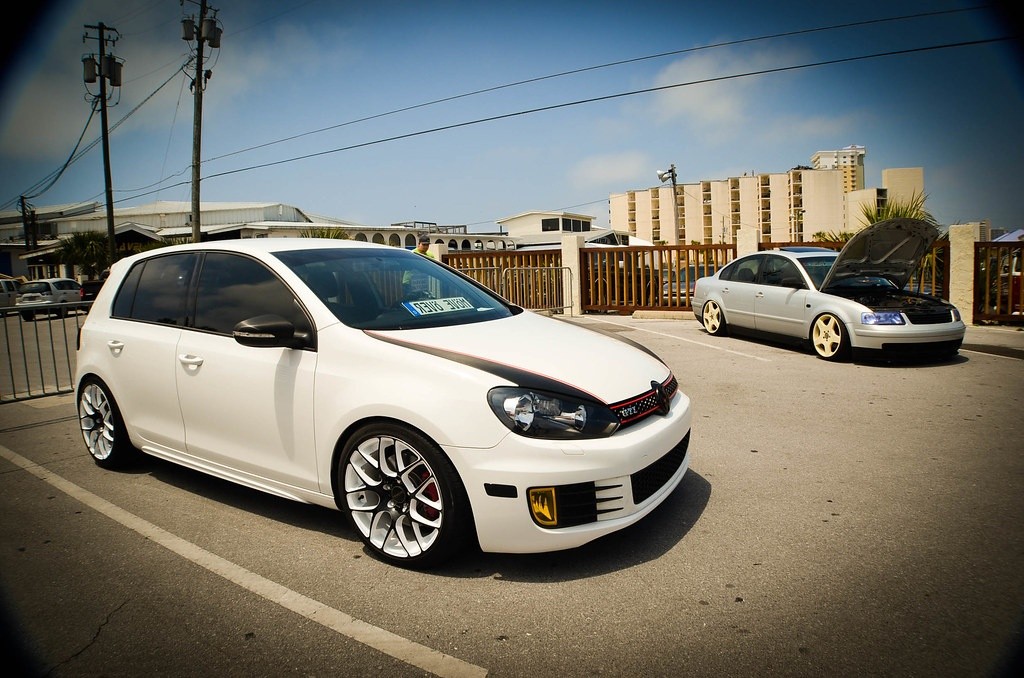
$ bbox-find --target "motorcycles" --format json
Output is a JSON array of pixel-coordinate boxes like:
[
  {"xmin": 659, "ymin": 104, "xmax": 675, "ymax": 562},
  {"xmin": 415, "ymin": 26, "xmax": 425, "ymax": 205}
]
[{"xmin": 992, "ymin": 248, "xmax": 1023, "ymax": 327}]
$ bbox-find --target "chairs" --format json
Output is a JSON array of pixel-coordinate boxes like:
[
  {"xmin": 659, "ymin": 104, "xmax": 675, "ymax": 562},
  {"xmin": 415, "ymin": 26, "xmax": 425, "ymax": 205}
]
[
  {"xmin": 738, "ymin": 268, "xmax": 755, "ymax": 283},
  {"xmin": 306, "ymin": 267, "xmax": 354, "ymax": 325}
]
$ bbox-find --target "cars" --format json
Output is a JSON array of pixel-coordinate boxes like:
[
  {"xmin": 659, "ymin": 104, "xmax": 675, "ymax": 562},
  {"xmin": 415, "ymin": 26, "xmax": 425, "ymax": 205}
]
[
  {"xmin": 74, "ymin": 235, "xmax": 695, "ymax": 575},
  {"xmin": 14, "ymin": 278, "xmax": 82, "ymax": 322},
  {"xmin": 0, "ymin": 278, "xmax": 22, "ymax": 317},
  {"xmin": 690, "ymin": 217, "xmax": 966, "ymax": 364}
]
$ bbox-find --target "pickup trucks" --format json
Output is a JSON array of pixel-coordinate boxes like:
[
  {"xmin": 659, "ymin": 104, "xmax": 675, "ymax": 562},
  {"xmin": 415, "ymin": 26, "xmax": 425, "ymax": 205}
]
[{"xmin": 80, "ymin": 268, "xmax": 111, "ymax": 313}]
